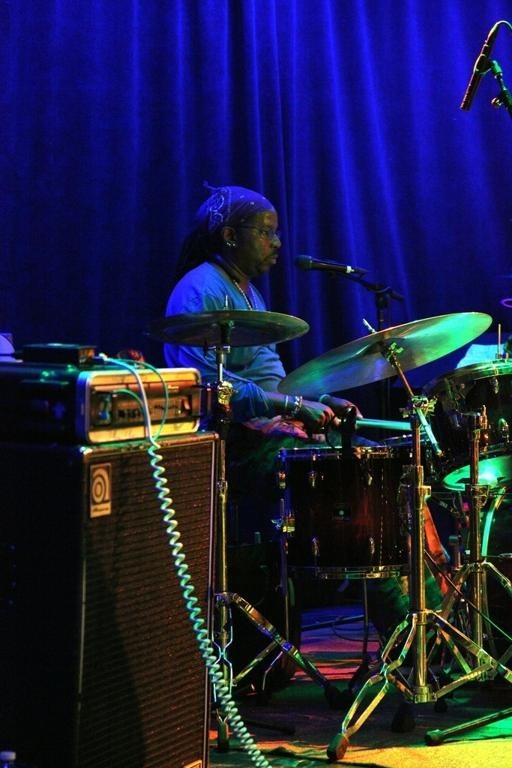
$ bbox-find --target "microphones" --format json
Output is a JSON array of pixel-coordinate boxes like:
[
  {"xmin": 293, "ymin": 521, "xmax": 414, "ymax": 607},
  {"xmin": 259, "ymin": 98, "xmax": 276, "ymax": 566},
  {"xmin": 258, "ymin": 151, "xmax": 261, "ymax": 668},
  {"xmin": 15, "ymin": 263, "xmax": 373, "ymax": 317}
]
[
  {"xmin": 294, "ymin": 253, "xmax": 352, "ymax": 281},
  {"xmin": 457, "ymin": 29, "xmax": 503, "ymax": 113}
]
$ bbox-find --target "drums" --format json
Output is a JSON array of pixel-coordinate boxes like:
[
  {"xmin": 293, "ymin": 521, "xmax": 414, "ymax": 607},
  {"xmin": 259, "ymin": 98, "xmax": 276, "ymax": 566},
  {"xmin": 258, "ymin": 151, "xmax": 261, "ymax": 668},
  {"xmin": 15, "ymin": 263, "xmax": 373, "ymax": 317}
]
[
  {"xmin": 442, "ymin": 455, "xmax": 511, "ymax": 653},
  {"xmin": 421, "ymin": 358, "xmax": 512, "ymax": 446},
  {"xmin": 277, "ymin": 445, "xmax": 412, "ymax": 582}
]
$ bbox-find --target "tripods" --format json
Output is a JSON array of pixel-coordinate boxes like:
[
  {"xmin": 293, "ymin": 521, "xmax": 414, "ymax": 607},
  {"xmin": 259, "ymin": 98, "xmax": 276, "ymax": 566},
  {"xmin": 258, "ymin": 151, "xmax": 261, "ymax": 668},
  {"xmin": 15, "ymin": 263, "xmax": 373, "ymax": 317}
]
[
  {"xmin": 329, "ymin": 423, "xmax": 511, "ymax": 762},
  {"xmin": 391, "ymin": 423, "xmax": 511, "ymax": 734},
  {"xmin": 213, "ymin": 432, "xmax": 343, "ymax": 751}
]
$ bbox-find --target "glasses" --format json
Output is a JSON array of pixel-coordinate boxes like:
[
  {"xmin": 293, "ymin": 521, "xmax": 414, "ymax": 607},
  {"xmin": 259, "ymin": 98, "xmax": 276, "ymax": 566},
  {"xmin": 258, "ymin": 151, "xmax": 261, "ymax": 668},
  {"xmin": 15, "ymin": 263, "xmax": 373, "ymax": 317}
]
[{"xmin": 223, "ymin": 222, "xmax": 283, "ymax": 241}]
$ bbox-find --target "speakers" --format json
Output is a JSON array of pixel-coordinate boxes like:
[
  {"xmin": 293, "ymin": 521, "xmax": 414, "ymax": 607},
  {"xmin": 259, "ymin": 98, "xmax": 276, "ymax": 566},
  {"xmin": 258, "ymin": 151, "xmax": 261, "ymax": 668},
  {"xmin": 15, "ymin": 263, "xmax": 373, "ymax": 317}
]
[{"xmin": 0, "ymin": 430, "xmax": 222, "ymax": 767}]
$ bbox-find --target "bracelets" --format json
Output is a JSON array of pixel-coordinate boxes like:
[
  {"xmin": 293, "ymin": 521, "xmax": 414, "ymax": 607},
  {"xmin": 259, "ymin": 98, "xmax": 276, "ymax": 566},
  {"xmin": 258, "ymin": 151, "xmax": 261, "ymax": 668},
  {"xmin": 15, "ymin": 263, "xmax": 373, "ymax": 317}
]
[
  {"xmin": 282, "ymin": 395, "xmax": 303, "ymax": 421},
  {"xmin": 319, "ymin": 393, "xmax": 330, "ymax": 404}
]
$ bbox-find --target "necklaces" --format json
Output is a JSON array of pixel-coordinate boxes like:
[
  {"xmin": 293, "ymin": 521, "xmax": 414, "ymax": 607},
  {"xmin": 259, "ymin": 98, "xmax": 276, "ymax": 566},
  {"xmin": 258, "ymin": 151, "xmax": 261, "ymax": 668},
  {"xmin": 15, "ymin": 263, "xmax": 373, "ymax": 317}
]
[{"xmin": 232, "ymin": 279, "xmax": 257, "ymax": 311}]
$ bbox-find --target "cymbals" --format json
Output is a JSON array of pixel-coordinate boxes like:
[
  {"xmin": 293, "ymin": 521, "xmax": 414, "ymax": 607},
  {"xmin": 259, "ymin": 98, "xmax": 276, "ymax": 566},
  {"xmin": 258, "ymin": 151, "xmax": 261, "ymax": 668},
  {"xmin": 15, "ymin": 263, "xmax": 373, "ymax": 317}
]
[
  {"xmin": 277, "ymin": 312, "xmax": 492, "ymax": 395},
  {"xmin": 149, "ymin": 309, "xmax": 309, "ymax": 348}
]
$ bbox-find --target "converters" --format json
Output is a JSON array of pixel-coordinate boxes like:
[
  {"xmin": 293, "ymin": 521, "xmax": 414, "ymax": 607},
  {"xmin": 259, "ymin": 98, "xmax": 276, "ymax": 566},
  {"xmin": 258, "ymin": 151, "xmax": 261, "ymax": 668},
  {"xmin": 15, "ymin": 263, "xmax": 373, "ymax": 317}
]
[{"xmin": 24, "ymin": 340, "xmax": 99, "ymax": 368}]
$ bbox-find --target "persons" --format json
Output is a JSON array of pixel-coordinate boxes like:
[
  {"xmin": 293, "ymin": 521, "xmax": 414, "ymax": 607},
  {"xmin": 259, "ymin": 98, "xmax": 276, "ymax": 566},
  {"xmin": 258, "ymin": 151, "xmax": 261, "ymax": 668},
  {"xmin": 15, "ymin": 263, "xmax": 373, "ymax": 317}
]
[{"xmin": 161, "ymin": 185, "xmax": 467, "ymax": 690}]
